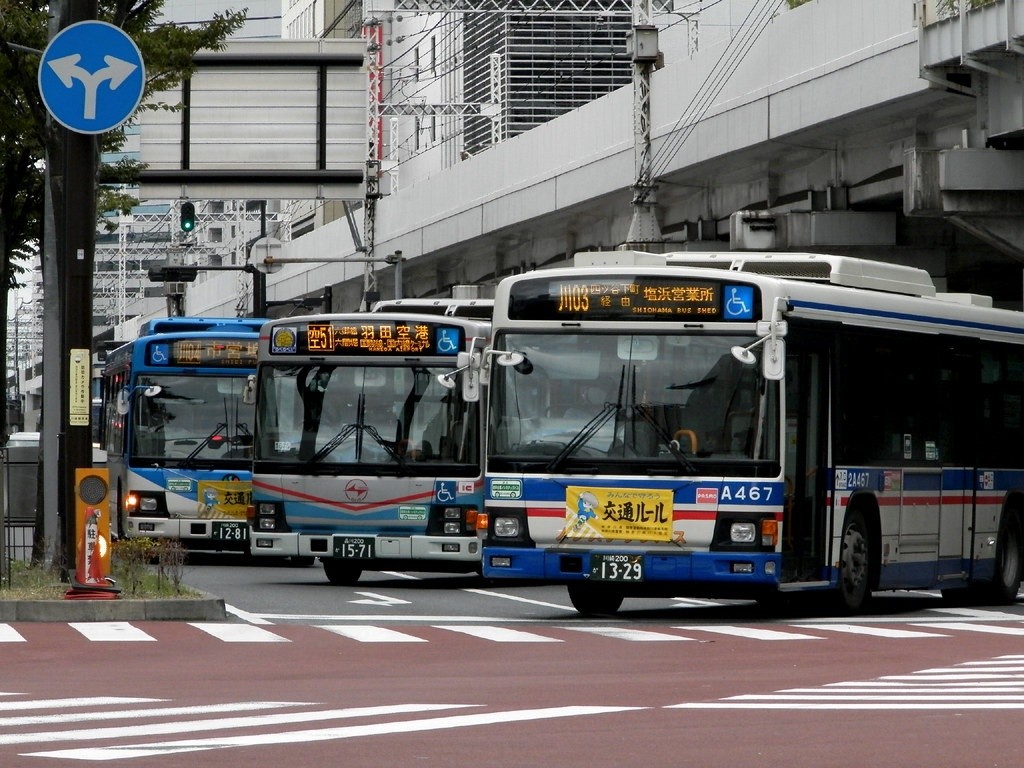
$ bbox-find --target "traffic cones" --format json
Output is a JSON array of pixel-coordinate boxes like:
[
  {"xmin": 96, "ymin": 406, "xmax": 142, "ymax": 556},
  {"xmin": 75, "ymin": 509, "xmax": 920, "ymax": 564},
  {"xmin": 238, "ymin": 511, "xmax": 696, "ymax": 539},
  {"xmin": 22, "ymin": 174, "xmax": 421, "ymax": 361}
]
[{"xmin": 65, "ymin": 506, "xmax": 117, "ymax": 599}]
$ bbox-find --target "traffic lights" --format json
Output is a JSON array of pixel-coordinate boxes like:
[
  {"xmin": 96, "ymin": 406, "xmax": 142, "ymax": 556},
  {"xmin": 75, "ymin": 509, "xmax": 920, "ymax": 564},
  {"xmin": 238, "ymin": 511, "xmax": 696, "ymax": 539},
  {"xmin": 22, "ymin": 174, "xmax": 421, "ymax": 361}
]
[{"xmin": 180, "ymin": 202, "xmax": 195, "ymax": 230}]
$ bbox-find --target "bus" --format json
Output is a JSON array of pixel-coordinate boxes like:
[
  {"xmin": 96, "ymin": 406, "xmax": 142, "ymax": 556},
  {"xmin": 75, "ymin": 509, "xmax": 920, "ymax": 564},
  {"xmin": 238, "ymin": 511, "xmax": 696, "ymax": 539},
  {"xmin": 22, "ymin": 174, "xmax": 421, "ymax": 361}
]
[
  {"xmin": 251, "ymin": 295, "xmax": 502, "ymax": 586},
  {"xmin": 97, "ymin": 316, "xmax": 273, "ymax": 556},
  {"xmin": 478, "ymin": 240, "xmax": 1024, "ymax": 617}
]
